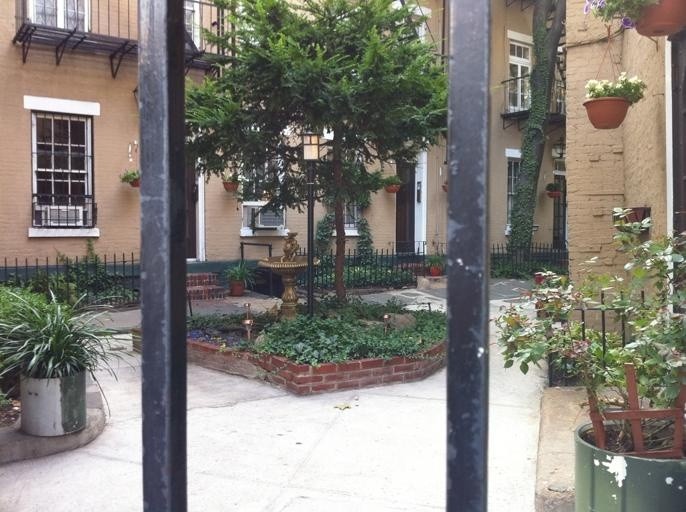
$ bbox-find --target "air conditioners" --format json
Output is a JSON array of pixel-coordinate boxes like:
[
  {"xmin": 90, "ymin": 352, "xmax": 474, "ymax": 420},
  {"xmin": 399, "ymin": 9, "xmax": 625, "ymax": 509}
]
[
  {"xmin": 41, "ymin": 206, "xmax": 83, "ymax": 227},
  {"xmin": 249, "ymin": 206, "xmax": 286, "ymax": 229}
]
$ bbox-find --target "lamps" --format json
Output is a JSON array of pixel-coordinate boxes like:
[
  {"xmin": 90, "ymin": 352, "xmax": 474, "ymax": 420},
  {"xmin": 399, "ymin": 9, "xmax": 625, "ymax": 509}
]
[{"xmin": 551, "ymin": 137, "xmax": 565, "ymax": 160}]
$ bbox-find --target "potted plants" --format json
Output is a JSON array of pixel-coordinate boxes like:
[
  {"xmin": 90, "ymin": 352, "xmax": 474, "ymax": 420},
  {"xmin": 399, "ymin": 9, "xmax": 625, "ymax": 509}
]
[
  {"xmin": 497, "ymin": 209, "xmax": 686, "ymax": 512},
  {"xmin": 545, "ymin": 183, "xmax": 561, "ymax": 198},
  {"xmin": 121, "ymin": 171, "xmax": 140, "ymax": 187},
  {"xmin": 384, "ymin": 175, "xmax": 401, "ymax": 193},
  {"xmin": 429, "ymin": 256, "xmax": 444, "ymax": 276},
  {"xmin": 0, "ymin": 287, "xmax": 141, "ymax": 436},
  {"xmin": 223, "ymin": 260, "xmax": 254, "ymax": 296},
  {"xmin": 221, "ymin": 173, "xmax": 241, "ymax": 192}
]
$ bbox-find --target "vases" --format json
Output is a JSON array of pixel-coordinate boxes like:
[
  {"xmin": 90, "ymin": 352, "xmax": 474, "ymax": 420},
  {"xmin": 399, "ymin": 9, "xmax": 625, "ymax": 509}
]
[
  {"xmin": 582, "ymin": 97, "xmax": 633, "ymax": 129},
  {"xmin": 634, "ymin": 0, "xmax": 686, "ymax": 36}
]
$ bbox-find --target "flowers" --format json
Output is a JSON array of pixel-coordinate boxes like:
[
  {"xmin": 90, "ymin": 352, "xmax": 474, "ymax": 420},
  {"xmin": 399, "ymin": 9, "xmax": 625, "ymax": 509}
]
[
  {"xmin": 584, "ymin": 0, "xmax": 661, "ymax": 29},
  {"xmin": 585, "ymin": 72, "xmax": 648, "ymax": 103}
]
